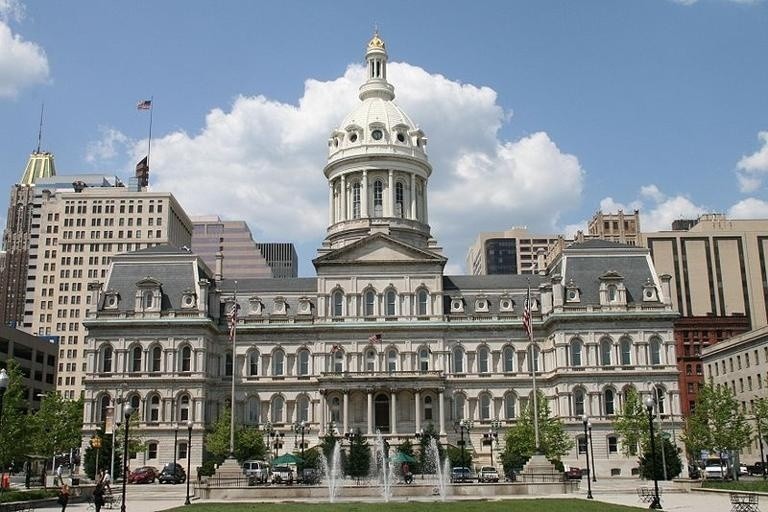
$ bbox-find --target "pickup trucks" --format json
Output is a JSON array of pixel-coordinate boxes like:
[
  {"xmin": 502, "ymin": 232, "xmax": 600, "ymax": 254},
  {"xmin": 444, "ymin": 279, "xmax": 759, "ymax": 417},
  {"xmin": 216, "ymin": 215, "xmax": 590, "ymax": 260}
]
[{"xmin": 452, "ymin": 465, "xmax": 499, "ymax": 483}]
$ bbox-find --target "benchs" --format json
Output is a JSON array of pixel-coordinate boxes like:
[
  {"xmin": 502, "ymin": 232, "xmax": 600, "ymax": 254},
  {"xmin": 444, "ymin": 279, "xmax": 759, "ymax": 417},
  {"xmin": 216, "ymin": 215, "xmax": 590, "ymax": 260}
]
[
  {"xmin": 636, "ymin": 485, "xmax": 663, "ymax": 504},
  {"xmin": 729, "ymin": 490, "xmax": 761, "ymax": 512}
]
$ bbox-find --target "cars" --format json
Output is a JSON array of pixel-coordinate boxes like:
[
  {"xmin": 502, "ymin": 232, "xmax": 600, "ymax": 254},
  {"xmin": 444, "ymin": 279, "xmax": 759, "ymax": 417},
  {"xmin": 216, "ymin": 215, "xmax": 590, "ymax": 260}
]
[
  {"xmin": 243, "ymin": 460, "xmax": 323, "ymax": 484},
  {"xmin": 128, "ymin": 463, "xmax": 186, "ymax": 484},
  {"xmin": 688, "ymin": 456, "xmax": 768, "ymax": 479},
  {"xmin": 565, "ymin": 467, "xmax": 582, "ymax": 479}
]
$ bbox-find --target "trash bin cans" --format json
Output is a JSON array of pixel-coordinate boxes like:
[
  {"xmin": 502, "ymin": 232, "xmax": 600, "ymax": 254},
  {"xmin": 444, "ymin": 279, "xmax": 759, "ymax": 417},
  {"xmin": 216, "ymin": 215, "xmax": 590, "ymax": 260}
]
[{"xmin": 72, "ymin": 478, "xmax": 79, "ymax": 485}]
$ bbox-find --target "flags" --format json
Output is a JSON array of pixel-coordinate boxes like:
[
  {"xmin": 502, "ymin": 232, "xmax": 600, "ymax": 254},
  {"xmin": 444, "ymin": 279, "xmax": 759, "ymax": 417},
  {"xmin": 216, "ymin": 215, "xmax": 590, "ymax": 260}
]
[
  {"xmin": 523, "ymin": 284, "xmax": 531, "ymax": 339},
  {"xmin": 370, "ymin": 333, "xmax": 381, "ymax": 344},
  {"xmin": 137, "ymin": 100, "xmax": 151, "ymax": 109},
  {"xmin": 229, "ymin": 288, "xmax": 234, "ymax": 346}
]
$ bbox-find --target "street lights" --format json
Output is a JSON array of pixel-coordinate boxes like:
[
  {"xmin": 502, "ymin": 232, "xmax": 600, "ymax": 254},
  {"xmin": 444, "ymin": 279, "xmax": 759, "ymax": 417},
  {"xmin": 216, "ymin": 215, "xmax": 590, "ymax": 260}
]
[
  {"xmin": 645, "ymin": 397, "xmax": 661, "ymax": 510},
  {"xmin": 300, "ymin": 419, "xmax": 306, "ymax": 481},
  {"xmin": 489, "ymin": 428, "xmax": 493, "ymax": 466},
  {"xmin": 582, "ymin": 414, "xmax": 596, "ymax": 499},
  {"xmin": 349, "ymin": 428, "xmax": 354, "ymax": 479},
  {"xmin": 173, "ymin": 423, "xmax": 179, "ymax": 484},
  {"xmin": 459, "ymin": 419, "xmax": 466, "ymax": 483},
  {"xmin": 0, "ymin": 368, "xmax": 9, "ymax": 413},
  {"xmin": 185, "ymin": 420, "xmax": 193, "ymax": 505},
  {"xmin": 121, "ymin": 402, "xmax": 134, "ymax": 512}
]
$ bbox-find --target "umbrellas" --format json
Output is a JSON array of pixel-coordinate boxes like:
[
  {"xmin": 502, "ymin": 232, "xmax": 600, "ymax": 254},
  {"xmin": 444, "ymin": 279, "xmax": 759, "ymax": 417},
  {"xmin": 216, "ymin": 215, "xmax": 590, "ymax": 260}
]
[
  {"xmin": 388, "ymin": 453, "xmax": 421, "ymax": 465},
  {"xmin": 273, "ymin": 453, "xmax": 304, "ymax": 466}
]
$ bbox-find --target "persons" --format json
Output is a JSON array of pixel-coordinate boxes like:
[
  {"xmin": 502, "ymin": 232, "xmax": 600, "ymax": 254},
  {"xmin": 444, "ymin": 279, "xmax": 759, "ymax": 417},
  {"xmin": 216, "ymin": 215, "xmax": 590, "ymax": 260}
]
[
  {"xmin": 60, "ymin": 483, "xmax": 70, "ymax": 512},
  {"xmin": 2, "ymin": 471, "xmax": 10, "ymax": 489},
  {"xmin": 93, "ymin": 482, "xmax": 106, "ymax": 512},
  {"xmin": 402, "ymin": 462, "xmax": 411, "ymax": 483},
  {"xmin": 102, "ymin": 469, "xmax": 110, "ymax": 483}
]
